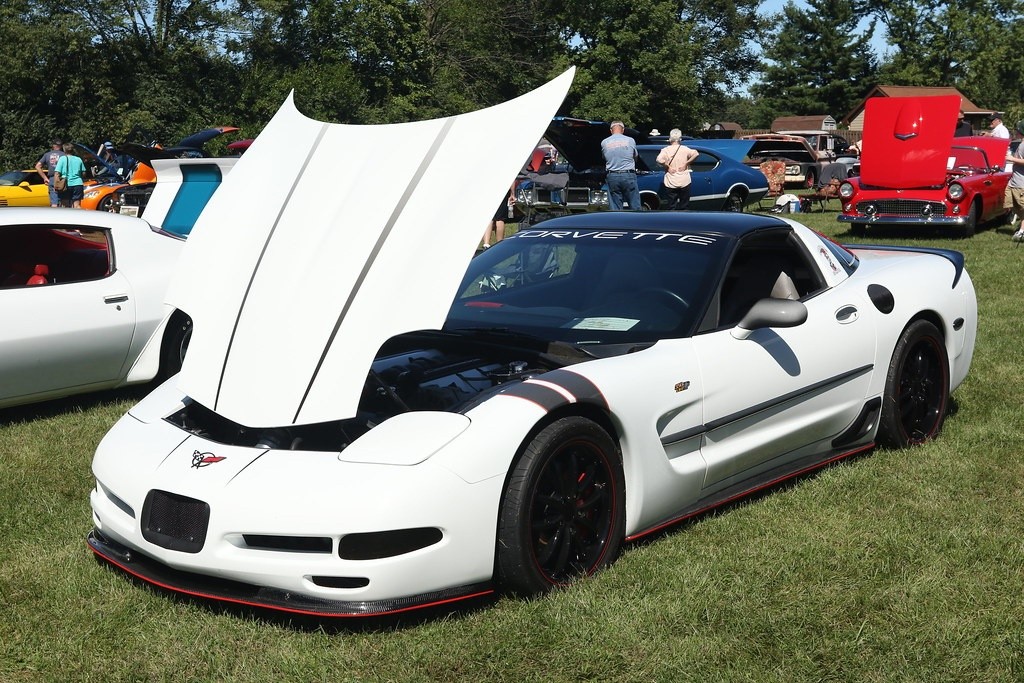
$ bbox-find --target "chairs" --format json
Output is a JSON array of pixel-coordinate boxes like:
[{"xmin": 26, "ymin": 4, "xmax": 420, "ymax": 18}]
[
  {"xmin": 718, "ymin": 240, "xmax": 800, "ymax": 325},
  {"xmin": 799, "ymin": 163, "xmax": 846, "ymax": 214},
  {"xmin": 0, "ymin": 261, "xmax": 49, "ymax": 286},
  {"xmin": 757, "ymin": 161, "xmax": 786, "ymax": 211}
]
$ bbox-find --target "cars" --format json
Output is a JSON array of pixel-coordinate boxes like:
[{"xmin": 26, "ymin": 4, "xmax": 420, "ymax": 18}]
[
  {"xmin": 836, "ymin": 96, "xmax": 1018, "ymax": 236},
  {"xmin": 514, "ymin": 116, "xmax": 771, "ymax": 213},
  {"xmin": 748, "ymin": 129, "xmax": 862, "ymax": 189}
]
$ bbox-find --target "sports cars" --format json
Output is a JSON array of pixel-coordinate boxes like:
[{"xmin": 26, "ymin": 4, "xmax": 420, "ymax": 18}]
[
  {"xmin": 0, "ymin": 168, "xmax": 51, "ymax": 208},
  {"xmin": 78, "ymin": 139, "xmax": 164, "ymax": 214},
  {"xmin": 1, "ymin": 158, "xmax": 243, "ymax": 416},
  {"xmin": 88, "ymin": 65, "xmax": 983, "ymax": 625}
]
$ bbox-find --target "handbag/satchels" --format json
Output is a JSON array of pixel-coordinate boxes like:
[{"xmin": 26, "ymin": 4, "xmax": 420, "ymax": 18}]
[
  {"xmin": 658, "ymin": 182, "xmax": 667, "ymax": 200},
  {"xmin": 54, "ymin": 178, "xmax": 67, "ymax": 192}
]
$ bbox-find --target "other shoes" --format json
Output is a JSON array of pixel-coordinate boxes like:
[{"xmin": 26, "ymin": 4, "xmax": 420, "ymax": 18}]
[
  {"xmin": 482, "ymin": 245, "xmax": 490, "ymax": 252},
  {"xmin": 1013, "ymin": 230, "xmax": 1024, "ymax": 240}
]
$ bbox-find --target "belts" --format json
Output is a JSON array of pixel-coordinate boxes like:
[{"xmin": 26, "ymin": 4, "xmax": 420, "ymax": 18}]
[{"xmin": 606, "ymin": 170, "xmax": 635, "ymax": 174}]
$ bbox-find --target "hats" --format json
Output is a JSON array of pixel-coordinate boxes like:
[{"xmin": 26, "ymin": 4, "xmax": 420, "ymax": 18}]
[
  {"xmin": 105, "ymin": 142, "xmax": 113, "ymax": 149},
  {"xmin": 987, "ymin": 112, "xmax": 1001, "ymax": 121},
  {"xmin": 52, "ymin": 139, "xmax": 63, "ymax": 146},
  {"xmin": 1014, "ymin": 118, "xmax": 1024, "ymax": 135}
]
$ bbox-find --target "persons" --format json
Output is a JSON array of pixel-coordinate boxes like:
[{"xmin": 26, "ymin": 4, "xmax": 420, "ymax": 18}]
[
  {"xmin": 601, "ymin": 120, "xmax": 642, "ymax": 212},
  {"xmin": 35, "ymin": 139, "xmax": 87, "ymax": 209},
  {"xmin": 103, "ymin": 141, "xmax": 117, "ymax": 164},
  {"xmin": 1003, "ymin": 119, "xmax": 1024, "ymax": 242},
  {"xmin": 954, "ymin": 109, "xmax": 973, "ymax": 137},
  {"xmin": 980, "ymin": 111, "xmax": 1010, "ymax": 139},
  {"xmin": 656, "ymin": 129, "xmax": 700, "ymax": 211},
  {"xmin": 482, "ymin": 181, "xmax": 516, "ymax": 247}
]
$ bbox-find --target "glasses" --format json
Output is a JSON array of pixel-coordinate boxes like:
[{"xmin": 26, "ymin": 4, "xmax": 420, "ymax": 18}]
[{"xmin": 958, "ymin": 117, "xmax": 965, "ymax": 121}]
